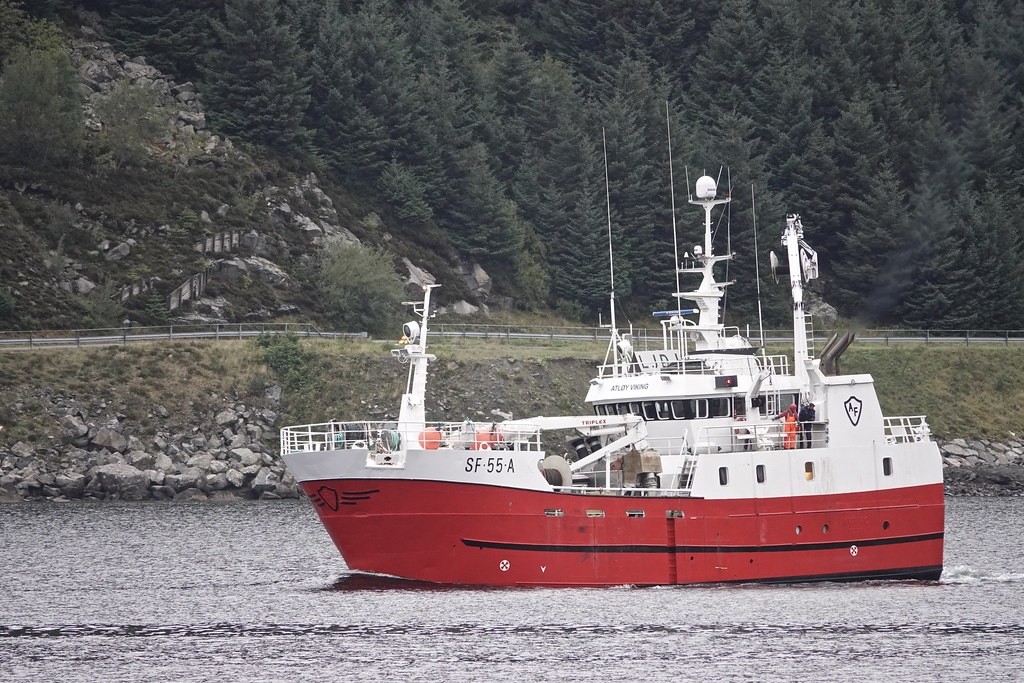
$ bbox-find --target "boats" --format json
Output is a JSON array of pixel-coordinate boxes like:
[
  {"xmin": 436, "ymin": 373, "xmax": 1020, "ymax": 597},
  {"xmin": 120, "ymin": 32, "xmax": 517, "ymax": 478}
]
[{"xmin": 279, "ymin": 99, "xmax": 949, "ymax": 586}]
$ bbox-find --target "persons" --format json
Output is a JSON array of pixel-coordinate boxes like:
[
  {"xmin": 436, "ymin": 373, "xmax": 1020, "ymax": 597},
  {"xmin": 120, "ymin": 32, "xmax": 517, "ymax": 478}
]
[
  {"xmin": 770, "ymin": 403, "xmax": 800, "ymax": 450},
  {"xmin": 799, "ymin": 403, "xmax": 816, "ymax": 449}
]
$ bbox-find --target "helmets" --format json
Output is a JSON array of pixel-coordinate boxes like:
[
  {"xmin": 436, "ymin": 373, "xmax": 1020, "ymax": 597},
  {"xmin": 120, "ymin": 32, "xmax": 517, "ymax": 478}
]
[{"xmin": 790, "ymin": 404, "xmax": 797, "ymax": 409}]
